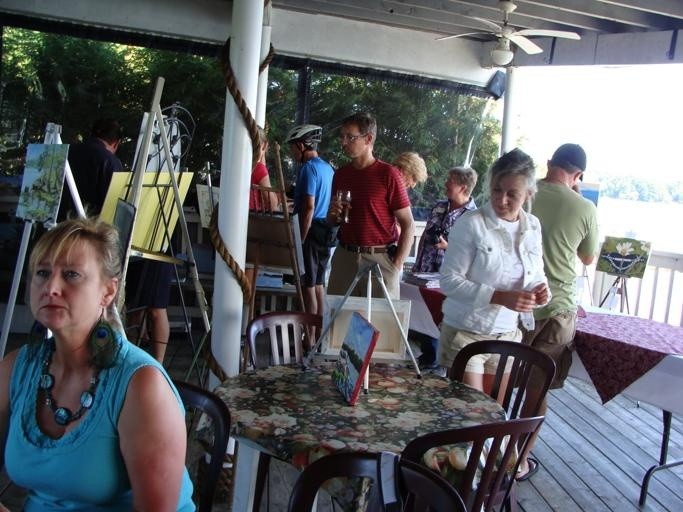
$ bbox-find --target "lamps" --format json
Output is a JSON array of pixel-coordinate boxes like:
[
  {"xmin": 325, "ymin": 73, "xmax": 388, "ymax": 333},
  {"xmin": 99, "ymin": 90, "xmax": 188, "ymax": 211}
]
[{"xmin": 489, "ymin": 39, "xmax": 515, "ymax": 66}]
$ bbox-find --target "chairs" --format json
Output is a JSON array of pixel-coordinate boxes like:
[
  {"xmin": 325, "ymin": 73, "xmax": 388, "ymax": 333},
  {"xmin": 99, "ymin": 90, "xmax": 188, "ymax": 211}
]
[
  {"xmin": 400, "ymin": 414, "xmax": 544, "ymax": 512},
  {"xmin": 244, "ymin": 311, "xmax": 321, "ymax": 371},
  {"xmin": 449, "ymin": 340, "xmax": 556, "ymax": 512},
  {"xmin": 171, "ymin": 380, "xmax": 230, "ymax": 511},
  {"xmin": 286, "ymin": 450, "xmax": 466, "ymax": 512}
]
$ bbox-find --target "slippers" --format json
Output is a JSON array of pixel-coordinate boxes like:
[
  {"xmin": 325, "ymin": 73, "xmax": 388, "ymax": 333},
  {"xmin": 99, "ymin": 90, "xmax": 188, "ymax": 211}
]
[{"xmin": 515, "ymin": 457, "xmax": 539, "ymax": 481}]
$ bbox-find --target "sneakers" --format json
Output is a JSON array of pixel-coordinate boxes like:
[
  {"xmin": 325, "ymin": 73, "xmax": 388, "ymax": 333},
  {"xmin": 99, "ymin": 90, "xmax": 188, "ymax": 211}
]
[
  {"xmin": 430, "ymin": 367, "xmax": 448, "ymax": 378},
  {"xmin": 407, "ymin": 354, "xmax": 438, "ymax": 370}
]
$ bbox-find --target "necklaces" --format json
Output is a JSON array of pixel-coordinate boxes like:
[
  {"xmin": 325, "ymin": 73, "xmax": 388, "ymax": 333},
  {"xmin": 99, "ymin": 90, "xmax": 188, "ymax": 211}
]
[{"xmin": 38, "ymin": 337, "xmax": 102, "ymax": 425}]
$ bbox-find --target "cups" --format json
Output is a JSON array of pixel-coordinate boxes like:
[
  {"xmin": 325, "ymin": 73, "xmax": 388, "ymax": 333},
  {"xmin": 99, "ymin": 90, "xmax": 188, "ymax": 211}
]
[{"xmin": 335, "ymin": 190, "xmax": 352, "ymax": 225}]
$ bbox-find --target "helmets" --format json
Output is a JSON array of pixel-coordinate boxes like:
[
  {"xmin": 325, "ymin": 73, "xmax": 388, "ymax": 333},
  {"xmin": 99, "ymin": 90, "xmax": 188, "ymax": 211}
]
[{"xmin": 284, "ymin": 124, "xmax": 323, "ymax": 143}]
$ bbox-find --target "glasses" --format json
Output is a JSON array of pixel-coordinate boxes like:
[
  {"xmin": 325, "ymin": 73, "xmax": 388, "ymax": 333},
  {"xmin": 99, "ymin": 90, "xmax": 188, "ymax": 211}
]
[{"xmin": 339, "ymin": 131, "xmax": 371, "ymax": 142}]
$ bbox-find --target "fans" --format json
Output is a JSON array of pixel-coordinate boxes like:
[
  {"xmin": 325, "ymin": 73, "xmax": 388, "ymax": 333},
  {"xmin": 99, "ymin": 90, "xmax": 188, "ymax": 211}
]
[{"xmin": 434, "ymin": 0, "xmax": 581, "ymax": 55}]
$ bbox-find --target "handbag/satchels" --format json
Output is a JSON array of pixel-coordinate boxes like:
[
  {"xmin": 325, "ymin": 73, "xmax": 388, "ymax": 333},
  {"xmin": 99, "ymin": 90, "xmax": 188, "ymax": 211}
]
[{"xmin": 310, "ymin": 219, "xmax": 341, "ymax": 248}]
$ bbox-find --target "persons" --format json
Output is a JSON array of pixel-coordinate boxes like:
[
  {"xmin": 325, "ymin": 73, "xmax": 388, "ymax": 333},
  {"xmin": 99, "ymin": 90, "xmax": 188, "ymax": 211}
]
[
  {"xmin": 322, "ymin": 114, "xmax": 414, "ymax": 300},
  {"xmin": 0, "ymin": 218, "xmax": 197, "ymax": 512},
  {"xmin": 437, "ymin": 147, "xmax": 553, "ymax": 411},
  {"xmin": 59, "ymin": 115, "xmax": 123, "ymax": 219},
  {"xmin": 511, "ymin": 144, "xmax": 596, "ymax": 482},
  {"xmin": 282, "ymin": 124, "xmax": 338, "ymax": 355},
  {"xmin": 249, "ymin": 126, "xmax": 278, "ymax": 211},
  {"xmin": 391, "ymin": 152, "xmax": 428, "ymax": 189},
  {"xmin": 411, "ymin": 166, "xmax": 479, "ymax": 273},
  {"xmin": 125, "ymin": 227, "xmax": 180, "ymax": 366}
]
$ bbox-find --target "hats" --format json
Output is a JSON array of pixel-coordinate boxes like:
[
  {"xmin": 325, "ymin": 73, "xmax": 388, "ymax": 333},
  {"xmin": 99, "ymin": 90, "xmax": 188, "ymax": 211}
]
[{"xmin": 554, "ymin": 143, "xmax": 586, "ymax": 182}]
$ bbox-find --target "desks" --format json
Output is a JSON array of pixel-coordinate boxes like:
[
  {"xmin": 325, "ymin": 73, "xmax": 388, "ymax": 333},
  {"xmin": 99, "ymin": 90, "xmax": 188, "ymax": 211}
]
[
  {"xmin": 564, "ymin": 307, "xmax": 683, "ymax": 512},
  {"xmin": 214, "ymin": 361, "xmax": 521, "ymax": 511},
  {"xmin": 399, "ymin": 276, "xmax": 446, "ymax": 339}
]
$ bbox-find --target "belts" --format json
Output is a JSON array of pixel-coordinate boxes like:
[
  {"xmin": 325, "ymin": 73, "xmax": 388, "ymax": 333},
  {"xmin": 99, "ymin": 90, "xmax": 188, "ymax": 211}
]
[{"xmin": 338, "ymin": 242, "xmax": 388, "ymax": 253}]
